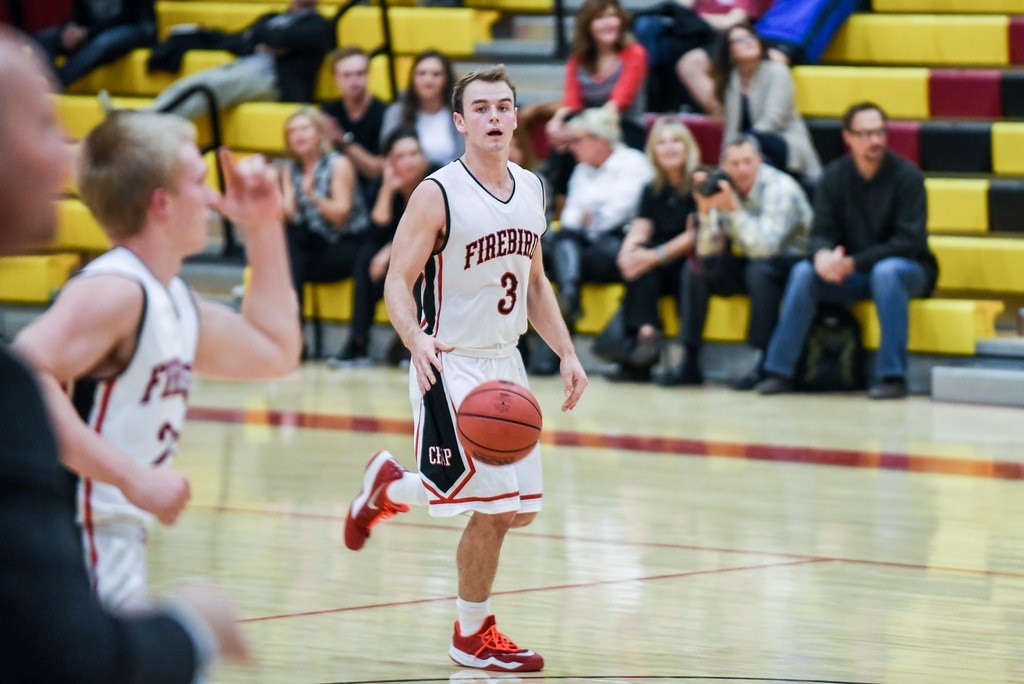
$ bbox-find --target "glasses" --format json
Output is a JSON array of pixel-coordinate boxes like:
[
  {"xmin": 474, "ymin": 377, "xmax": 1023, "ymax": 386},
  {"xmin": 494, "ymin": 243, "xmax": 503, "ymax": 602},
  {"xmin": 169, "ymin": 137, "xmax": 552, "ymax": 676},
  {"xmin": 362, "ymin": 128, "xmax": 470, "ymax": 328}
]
[
  {"xmin": 726, "ymin": 34, "xmax": 757, "ymax": 50},
  {"xmin": 846, "ymin": 127, "xmax": 886, "ymax": 138}
]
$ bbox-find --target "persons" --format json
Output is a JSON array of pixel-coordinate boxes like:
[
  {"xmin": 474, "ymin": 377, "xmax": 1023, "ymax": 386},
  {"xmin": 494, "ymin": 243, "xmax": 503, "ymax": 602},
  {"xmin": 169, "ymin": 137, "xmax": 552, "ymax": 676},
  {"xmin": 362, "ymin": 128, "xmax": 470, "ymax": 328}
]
[
  {"xmin": 0, "ymin": 24, "xmax": 259, "ymax": 683},
  {"xmin": 677, "ymin": 1, "xmax": 823, "ymax": 190},
  {"xmin": 345, "ymin": 57, "xmax": 590, "ymax": 672},
  {"xmin": 653, "ymin": 136, "xmax": 816, "ymax": 390},
  {"xmin": 601, "ymin": 115, "xmax": 701, "ymax": 384},
  {"xmin": 277, "ymin": 105, "xmax": 372, "ymax": 363},
  {"xmin": 527, "ymin": 106, "xmax": 658, "ymax": 377},
  {"xmin": 319, "ymin": 42, "xmax": 388, "ymax": 191},
  {"xmin": 334, "ymin": 122, "xmax": 452, "ymax": 367},
  {"xmin": 32, "ymin": 0, "xmax": 161, "ymax": 89},
  {"xmin": 756, "ymin": 101, "xmax": 941, "ymax": 399},
  {"xmin": 546, "ymin": 0, "xmax": 648, "ymax": 195},
  {"xmin": 8, "ymin": 108, "xmax": 302, "ymax": 613},
  {"xmin": 380, "ymin": 48, "xmax": 465, "ymax": 167},
  {"xmin": 147, "ymin": 0, "xmax": 338, "ymax": 121}
]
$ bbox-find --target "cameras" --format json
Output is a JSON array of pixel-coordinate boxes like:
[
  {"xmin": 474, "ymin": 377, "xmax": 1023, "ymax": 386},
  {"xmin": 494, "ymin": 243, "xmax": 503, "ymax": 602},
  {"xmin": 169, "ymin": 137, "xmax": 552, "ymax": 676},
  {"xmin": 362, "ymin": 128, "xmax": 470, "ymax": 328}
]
[{"xmin": 695, "ymin": 169, "xmax": 732, "ymax": 197}]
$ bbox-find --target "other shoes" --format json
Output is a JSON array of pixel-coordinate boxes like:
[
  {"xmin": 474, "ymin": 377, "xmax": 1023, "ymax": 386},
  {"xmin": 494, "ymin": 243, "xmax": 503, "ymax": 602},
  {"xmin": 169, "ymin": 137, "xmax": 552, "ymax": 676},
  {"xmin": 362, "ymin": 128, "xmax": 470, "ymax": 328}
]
[
  {"xmin": 323, "ymin": 338, "xmax": 373, "ymax": 367},
  {"xmin": 733, "ymin": 370, "xmax": 764, "ymax": 388},
  {"xmin": 752, "ymin": 375, "xmax": 789, "ymax": 394},
  {"xmin": 657, "ymin": 364, "xmax": 706, "ymax": 385},
  {"xmin": 628, "ymin": 333, "xmax": 665, "ymax": 365},
  {"xmin": 865, "ymin": 380, "xmax": 908, "ymax": 399}
]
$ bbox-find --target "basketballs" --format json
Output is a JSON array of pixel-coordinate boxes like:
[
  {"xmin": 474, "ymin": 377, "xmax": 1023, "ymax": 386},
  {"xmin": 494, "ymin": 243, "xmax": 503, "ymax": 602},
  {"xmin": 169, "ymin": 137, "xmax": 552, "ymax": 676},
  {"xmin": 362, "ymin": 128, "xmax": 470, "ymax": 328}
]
[{"xmin": 454, "ymin": 379, "xmax": 543, "ymax": 468}]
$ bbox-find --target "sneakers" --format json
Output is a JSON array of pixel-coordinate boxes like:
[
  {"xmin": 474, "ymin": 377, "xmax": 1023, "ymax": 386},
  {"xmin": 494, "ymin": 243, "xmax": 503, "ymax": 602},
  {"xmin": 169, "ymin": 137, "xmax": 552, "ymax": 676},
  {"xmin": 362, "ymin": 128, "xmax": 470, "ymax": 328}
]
[
  {"xmin": 340, "ymin": 448, "xmax": 411, "ymax": 552},
  {"xmin": 448, "ymin": 614, "xmax": 545, "ymax": 671}
]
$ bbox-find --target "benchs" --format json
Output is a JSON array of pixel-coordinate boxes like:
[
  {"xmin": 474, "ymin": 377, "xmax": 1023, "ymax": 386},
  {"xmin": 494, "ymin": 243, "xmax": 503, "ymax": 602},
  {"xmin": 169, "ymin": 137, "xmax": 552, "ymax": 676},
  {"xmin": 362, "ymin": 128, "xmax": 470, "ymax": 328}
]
[{"xmin": 0, "ymin": 0, "xmax": 1024, "ymax": 397}]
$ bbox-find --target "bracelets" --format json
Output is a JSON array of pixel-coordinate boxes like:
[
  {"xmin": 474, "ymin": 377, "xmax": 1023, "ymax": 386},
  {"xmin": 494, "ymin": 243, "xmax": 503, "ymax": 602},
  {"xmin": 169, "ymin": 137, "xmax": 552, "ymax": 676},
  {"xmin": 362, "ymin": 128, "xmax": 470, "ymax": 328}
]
[{"xmin": 331, "ymin": 131, "xmax": 355, "ymax": 149}]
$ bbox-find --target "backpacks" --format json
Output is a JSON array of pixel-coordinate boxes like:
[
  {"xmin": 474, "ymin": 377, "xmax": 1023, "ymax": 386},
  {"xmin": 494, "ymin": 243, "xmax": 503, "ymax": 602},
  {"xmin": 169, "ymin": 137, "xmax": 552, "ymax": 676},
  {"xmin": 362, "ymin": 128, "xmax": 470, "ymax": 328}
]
[{"xmin": 797, "ymin": 302, "xmax": 869, "ymax": 393}]
